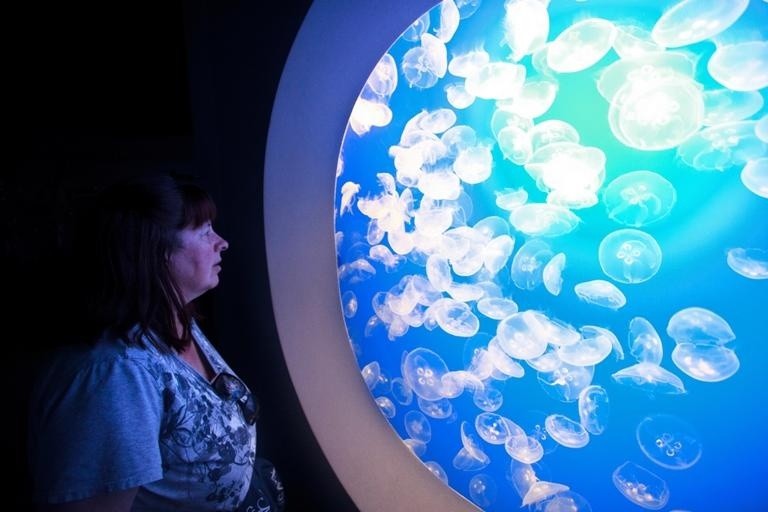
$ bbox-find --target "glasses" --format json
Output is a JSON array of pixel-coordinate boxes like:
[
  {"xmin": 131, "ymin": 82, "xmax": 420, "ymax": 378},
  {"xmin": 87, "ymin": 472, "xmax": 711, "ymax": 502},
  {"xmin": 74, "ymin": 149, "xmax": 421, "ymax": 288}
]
[{"xmin": 210, "ymin": 371, "xmax": 262, "ymax": 426}]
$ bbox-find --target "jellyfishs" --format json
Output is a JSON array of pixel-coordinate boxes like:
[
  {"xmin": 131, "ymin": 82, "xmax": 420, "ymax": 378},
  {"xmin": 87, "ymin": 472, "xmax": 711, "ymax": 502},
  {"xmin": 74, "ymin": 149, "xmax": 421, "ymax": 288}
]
[{"xmin": 334, "ymin": 0, "xmax": 766, "ymax": 512}]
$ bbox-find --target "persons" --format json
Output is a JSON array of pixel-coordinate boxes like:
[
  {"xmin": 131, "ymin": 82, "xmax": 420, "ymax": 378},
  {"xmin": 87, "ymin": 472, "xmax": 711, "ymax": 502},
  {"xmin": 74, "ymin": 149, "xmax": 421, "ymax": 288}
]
[{"xmin": 26, "ymin": 173, "xmax": 260, "ymax": 512}]
